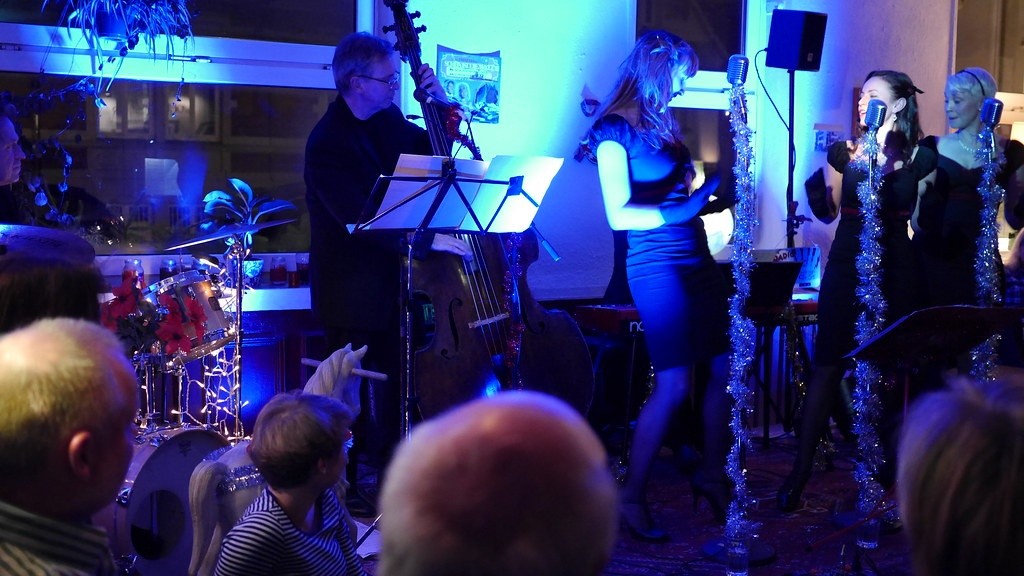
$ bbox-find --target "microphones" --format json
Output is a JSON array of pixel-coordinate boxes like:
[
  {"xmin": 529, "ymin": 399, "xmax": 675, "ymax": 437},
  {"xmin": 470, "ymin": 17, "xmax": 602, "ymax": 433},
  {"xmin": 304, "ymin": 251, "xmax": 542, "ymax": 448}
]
[
  {"xmin": 979, "ymin": 97, "xmax": 1004, "ymax": 161},
  {"xmin": 414, "ymin": 87, "xmax": 468, "ymax": 121},
  {"xmin": 865, "ymin": 99, "xmax": 887, "ymax": 167},
  {"xmin": 726, "ymin": 55, "xmax": 749, "ymax": 124}
]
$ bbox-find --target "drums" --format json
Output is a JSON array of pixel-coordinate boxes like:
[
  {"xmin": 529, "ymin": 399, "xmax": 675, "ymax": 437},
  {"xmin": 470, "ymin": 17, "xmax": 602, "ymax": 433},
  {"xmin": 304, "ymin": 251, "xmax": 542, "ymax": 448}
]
[
  {"xmin": 137, "ymin": 268, "xmax": 238, "ymax": 371},
  {"xmin": 95, "ymin": 289, "xmax": 164, "ymax": 369}
]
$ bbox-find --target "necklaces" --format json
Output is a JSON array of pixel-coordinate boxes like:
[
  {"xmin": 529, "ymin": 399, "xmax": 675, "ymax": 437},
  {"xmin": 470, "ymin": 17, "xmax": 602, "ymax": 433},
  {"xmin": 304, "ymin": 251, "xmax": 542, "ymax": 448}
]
[
  {"xmin": 861, "ymin": 141, "xmax": 887, "ymax": 159},
  {"xmin": 956, "ymin": 132, "xmax": 978, "ymax": 154}
]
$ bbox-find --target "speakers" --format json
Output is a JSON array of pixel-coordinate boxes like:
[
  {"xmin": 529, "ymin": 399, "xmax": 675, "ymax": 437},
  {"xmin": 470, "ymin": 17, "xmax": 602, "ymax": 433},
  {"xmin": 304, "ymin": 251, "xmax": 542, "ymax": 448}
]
[
  {"xmin": 576, "ymin": 305, "xmax": 702, "ymax": 452},
  {"xmin": 766, "ymin": 9, "xmax": 828, "ymax": 72}
]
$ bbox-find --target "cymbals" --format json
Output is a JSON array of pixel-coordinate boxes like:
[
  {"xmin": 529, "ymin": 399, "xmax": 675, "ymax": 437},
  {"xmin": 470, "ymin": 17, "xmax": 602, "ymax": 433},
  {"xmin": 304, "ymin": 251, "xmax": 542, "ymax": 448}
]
[{"xmin": 161, "ymin": 218, "xmax": 296, "ymax": 252}]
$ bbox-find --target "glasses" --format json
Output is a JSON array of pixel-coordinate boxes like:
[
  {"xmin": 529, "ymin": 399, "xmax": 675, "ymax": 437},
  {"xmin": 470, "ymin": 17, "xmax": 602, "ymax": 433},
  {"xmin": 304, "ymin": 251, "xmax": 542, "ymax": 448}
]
[{"xmin": 355, "ymin": 73, "xmax": 403, "ymax": 90}]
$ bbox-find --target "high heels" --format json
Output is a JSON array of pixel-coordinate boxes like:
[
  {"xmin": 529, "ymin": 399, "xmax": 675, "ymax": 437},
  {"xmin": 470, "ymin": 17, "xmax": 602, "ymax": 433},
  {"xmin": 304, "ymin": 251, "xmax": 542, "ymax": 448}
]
[
  {"xmin": 691, "ymin": 471, "xmax": 752, "ymax": 527},
  {"xmin": 619, "ymin": 492, "xmax": 670, "ymax": 543},
  {"xmin": 777, "ymin": 471, "xmax": 810, "ymax": 509}
]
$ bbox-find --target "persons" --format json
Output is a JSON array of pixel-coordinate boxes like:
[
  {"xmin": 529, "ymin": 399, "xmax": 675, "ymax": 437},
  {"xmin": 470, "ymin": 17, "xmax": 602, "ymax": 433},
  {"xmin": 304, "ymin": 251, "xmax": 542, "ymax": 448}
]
[
  {"xmin": 0, "ymin": 316, "xmax": 141, "ymax": 576},
  {"xmin": 0, "ymin": 227, "xmax": 104, "ymax": 331},
  {"xmin": 583, "ymin": 30, "xmax": 736, "ymax": 544},
  {"xmin": 375, "ymin": 389, "xmax": 617, "ymax": 576},
  {"xmin": 302, "ymin": 32, "xmax": 472, "ymax": 517},
  {"xmin": 214, "ymin": 388, "xmax": 376, "ymax": 576},
  {"xmin": 0, "ymin": 113, "xmax": 129, "ymax": 244},
  {"xmin": 777, "ymin": 66, "xmax": 1024, "ymax": 534},
  {"xmin": 898, "ymin": 362, "xmax": 1024, "ymax": 576}
]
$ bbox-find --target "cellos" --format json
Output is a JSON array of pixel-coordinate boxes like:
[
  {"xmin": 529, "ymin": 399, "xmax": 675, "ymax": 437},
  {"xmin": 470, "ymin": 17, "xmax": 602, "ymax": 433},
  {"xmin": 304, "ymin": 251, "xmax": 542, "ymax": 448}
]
[{"xmin": 377, "ymin": 0, "xmax": 599, "ymax": 428}]
